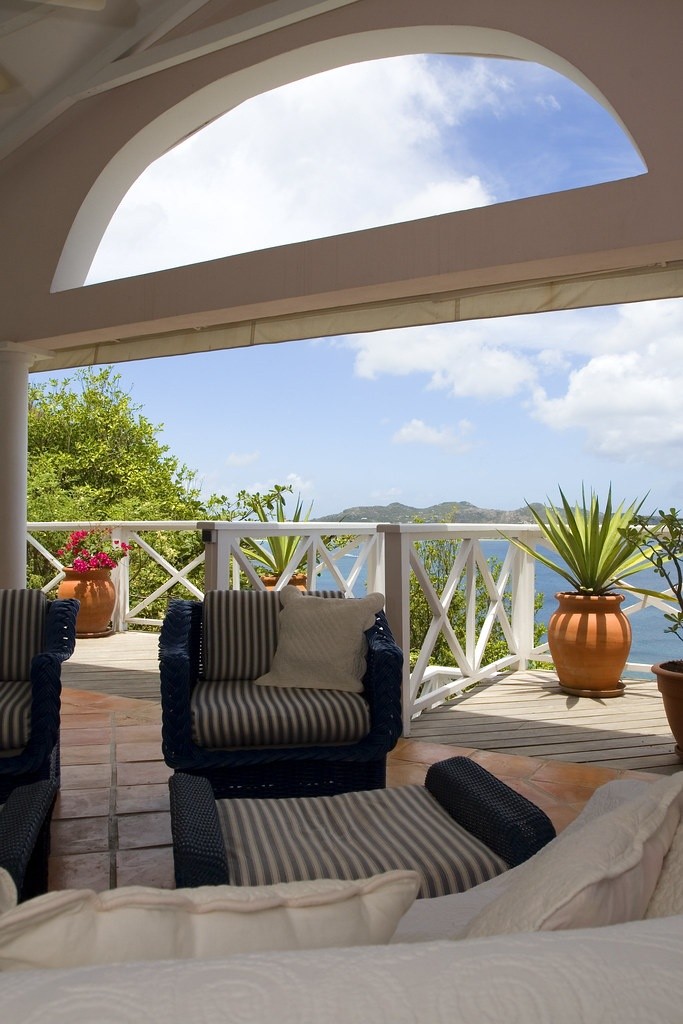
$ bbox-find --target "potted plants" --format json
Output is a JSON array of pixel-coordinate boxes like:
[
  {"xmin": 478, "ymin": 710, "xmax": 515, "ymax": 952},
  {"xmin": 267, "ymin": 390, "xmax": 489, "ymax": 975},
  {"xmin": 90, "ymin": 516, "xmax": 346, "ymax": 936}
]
[
  {"xmin": 616, "ymin": 507, "xmax": 683, "ymax": 758},
  {"xmin": 232, "ymin": 492, "xmax": 357, "ymax": 584},
  {"xmin": 490, "ymin": 478, "xmax": 682, "ymax": 697}
]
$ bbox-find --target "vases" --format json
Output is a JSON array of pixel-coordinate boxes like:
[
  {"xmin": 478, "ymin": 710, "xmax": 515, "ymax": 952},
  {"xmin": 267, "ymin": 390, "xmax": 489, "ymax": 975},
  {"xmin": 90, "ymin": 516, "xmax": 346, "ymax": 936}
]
[{"xmin": 57, "ymin": 567, "xmax": 118, "ymax": 637}]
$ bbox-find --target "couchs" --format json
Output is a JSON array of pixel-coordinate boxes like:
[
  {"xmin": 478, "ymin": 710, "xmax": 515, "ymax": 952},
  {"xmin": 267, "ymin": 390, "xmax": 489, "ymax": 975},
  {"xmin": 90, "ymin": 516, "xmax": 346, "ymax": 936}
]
[{"xmin": 0, "ymin": 775, "xmax": 681, "ymax": 1024}]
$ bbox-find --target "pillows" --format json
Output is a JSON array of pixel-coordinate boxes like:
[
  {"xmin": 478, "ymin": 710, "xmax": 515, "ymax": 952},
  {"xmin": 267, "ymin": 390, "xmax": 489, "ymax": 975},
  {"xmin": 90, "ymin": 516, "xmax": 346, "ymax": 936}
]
[
  {"xmin": 253, "ymin": 584, "xmax": 384, "ymax": 695},
  {"xmin": 460, "ymin": 770, "xmax": 683, "ymax": 944},
  {"xmin": 0, "ymin": 866, "xmax": 424, "ymax": 968}
]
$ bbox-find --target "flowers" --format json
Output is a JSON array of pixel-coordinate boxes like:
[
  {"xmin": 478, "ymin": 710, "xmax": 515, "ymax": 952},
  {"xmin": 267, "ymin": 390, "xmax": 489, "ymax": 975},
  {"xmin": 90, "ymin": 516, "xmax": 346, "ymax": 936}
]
[{"xmin": 55, "ymin": 529, "xmax": 136, "ymax": 573}]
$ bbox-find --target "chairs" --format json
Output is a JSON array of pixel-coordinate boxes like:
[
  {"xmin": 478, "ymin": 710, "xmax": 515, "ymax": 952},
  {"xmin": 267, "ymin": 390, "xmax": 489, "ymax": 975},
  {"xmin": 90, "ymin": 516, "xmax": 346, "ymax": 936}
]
[
  {"xmin": 158, "ymin": 586, "xmax": 405, "ymax": 800},
  {"xmin": 0, "ymin": 585, "xmax": 81, "ymax": 784},
  {"xmin": 163, "ymin": 751, "xmax": 560, "ymax": 902}
]
